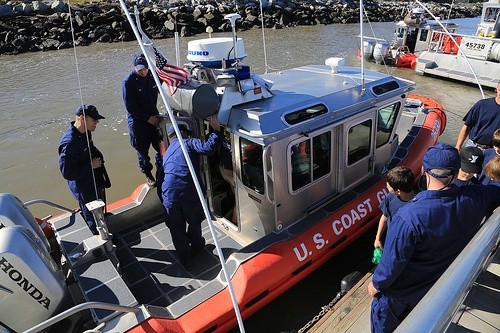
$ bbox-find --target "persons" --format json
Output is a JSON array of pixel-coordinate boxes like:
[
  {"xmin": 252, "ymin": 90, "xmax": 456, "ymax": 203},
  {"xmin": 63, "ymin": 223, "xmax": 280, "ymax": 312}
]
[
  {"xmin": 368, "ymin": 143, "xmax": 500, "ymax": 333},
  {"xmin": 57, "ymin": 104, "xmax": 118, "ymax": 244},
  {"xmin": 162, "ymin": 116, "xmax": 222, "ymax": 268},
  {"xmin": 120, "ymin": 53, "xmax": 164, "ymax": 186},
  {"xmin": 374, "ymin": 127, "xmax": 500, "ymax": 250}
]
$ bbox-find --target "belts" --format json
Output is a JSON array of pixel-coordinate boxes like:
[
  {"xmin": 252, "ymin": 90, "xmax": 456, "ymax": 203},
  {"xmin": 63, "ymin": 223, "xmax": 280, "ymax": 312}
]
[{"xmin": 473, "ymin": 141, "xmax": 488, "ymax": 149}]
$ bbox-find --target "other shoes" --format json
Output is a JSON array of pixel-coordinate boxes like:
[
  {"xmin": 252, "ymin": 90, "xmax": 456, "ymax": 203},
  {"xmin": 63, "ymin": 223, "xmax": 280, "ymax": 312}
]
[
  {"xmin": 191, "ymin": 237, "xmax": 205, "ymax": 257},
  {"xmin": 146, "ymin": 175, "xmax": 156, "ymax": 187},
  {"xmin": 179, "ymin": 246, "xmax": 190, "ymax": 265}
]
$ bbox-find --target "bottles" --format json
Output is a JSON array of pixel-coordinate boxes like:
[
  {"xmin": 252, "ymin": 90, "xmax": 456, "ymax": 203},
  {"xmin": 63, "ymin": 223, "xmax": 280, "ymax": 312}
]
[{"xmin": 372, "ymin": 247, "xmax": 383, "ymax": 264}]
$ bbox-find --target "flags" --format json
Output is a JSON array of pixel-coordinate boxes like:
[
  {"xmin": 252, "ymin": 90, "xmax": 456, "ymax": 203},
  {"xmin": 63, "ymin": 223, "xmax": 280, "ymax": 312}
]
[{"xmin": 136, "ymin": 15, "xmax": 191, "ymax": 96}]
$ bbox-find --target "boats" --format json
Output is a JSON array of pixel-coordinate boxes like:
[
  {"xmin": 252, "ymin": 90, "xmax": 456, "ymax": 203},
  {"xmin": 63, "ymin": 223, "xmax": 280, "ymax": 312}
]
[{"xmin": 0, "ymin": 0, "xmax": 500, "ymax": 333}]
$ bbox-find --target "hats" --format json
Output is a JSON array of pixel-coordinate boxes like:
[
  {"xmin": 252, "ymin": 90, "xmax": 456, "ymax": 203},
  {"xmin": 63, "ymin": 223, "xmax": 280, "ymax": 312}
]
[
  {"xmin": 459, "ymin": 146, "xmax": 484, "ymax": 174},
  {"xmin": 134, "ymin": 54, "xmax": 147, "ymax": 66},
  {"xmin": 167, "ymin": 121, "xmax": 191, "ymax": 137},
  {"xmin": 75, "ymin": 104, "xmax": 106, "ymax": 120},
  {"xmin": 422, "ymin": 143, "xmax": 461, "ymax": 178}
]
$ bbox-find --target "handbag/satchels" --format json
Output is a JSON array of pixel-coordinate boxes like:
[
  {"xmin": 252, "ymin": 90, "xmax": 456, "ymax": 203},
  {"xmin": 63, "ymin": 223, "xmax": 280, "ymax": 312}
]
[
  {"xmin": 395, "ymin": 53, "xmax": 417, "ymax": 67},
  {"xmin": 381, "ymin": 54, "xmax": 392, "ymax": 65}
]
